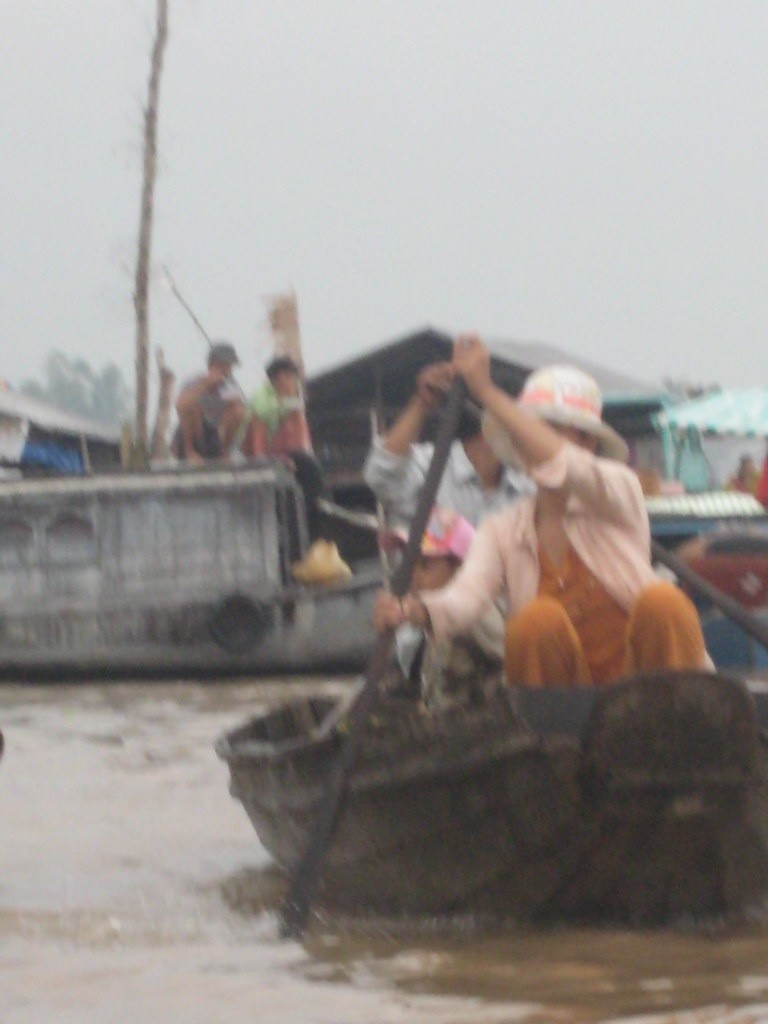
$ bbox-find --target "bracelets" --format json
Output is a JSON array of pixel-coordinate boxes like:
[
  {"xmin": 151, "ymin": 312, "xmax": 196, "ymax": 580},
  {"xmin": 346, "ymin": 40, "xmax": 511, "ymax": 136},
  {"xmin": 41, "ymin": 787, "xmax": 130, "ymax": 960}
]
[{"xmin": 397, "ymin": 597, "xmax": 405, "ymax": 612}]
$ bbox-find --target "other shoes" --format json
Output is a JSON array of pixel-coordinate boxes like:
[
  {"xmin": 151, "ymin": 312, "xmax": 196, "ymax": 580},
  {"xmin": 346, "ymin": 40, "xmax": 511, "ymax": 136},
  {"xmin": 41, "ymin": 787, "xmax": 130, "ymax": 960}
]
[{"xmin": 297, "ymin": 539, "xmax": 355, "ymax": 582}]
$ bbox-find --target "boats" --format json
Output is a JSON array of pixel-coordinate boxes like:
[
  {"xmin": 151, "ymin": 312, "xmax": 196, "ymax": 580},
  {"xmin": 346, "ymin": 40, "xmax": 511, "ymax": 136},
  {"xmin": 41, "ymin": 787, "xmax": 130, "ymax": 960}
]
[
  {"xmin": 659, "ymin": 515, "xmax": 768, "ymax": 679},
  {"xmin": 0, "ymin": 447, "xmax": 401, "ymax": 681},
  {"xmin": 213, "ymin": 660, "xmax": 768, "ymax": 933}
]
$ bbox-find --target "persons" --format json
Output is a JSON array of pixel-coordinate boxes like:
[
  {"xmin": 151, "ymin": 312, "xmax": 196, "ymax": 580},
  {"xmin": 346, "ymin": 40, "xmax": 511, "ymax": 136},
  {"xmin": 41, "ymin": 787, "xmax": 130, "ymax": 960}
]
[
  {"xmin": 167, "ymin": 343, "xmax": 249, "ymax": 467},
  {"xmin": 373, "ymin": 334, "xmax": 718, "ymax": 689},
  {"xmin": 363, "ymin": 361, "xmax": 537, "ymax": 678},
  {"xmin": 239, "ymin": 356, "xmax": 323, "ymax": 528},
  {"xmin": 726, "ymin": 450, "xmax": 768, "ymax": 504}
]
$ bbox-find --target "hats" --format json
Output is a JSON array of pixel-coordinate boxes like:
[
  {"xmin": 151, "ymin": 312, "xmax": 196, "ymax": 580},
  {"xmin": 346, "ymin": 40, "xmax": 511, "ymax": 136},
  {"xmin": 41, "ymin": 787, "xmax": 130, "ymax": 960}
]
[
  {"xmin": 479, "ymin": 364, "xmax": 630, "ymax": 474},
  {"xmin": 393, "ymin": 508, "xmax": 477, "ymax": 558},
  {"xmin": 208, "ymin": 345, "xmax": 241, "ymax": 365}
]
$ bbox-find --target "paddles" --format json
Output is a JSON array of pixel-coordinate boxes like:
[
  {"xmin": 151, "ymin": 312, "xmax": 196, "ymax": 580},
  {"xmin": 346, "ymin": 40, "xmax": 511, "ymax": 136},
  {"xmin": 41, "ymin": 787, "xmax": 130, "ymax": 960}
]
[
  {"xmin": 423, "ymin": 381, "xmax": 768, "ymax": 654},
  {"xmin": 274, "ymin": 334, "xmax": 476, "ymax": 947}
]
